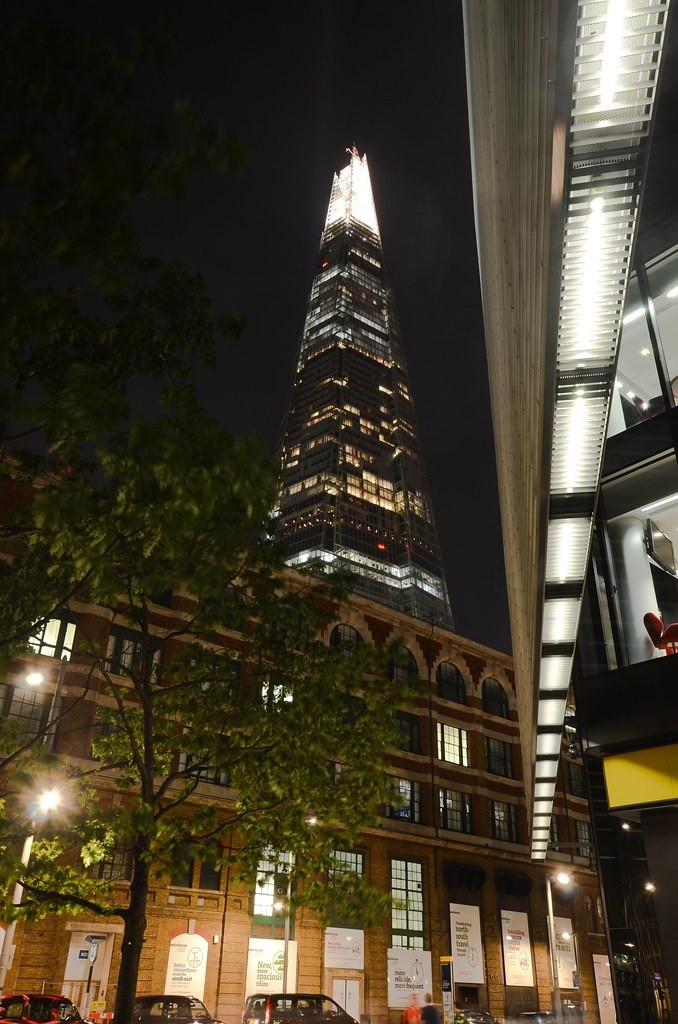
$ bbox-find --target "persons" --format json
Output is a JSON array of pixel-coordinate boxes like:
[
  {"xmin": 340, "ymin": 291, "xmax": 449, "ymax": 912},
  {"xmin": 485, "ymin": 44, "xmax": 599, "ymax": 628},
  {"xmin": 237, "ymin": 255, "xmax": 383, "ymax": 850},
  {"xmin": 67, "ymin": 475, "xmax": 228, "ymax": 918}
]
[
  {"xmin": 403, "ymin": 993, "xmax": 425, "ymax": 1024},
  {"xmin": 422, "ymin": 993, "xmax": 440, "ymax": 1024}
]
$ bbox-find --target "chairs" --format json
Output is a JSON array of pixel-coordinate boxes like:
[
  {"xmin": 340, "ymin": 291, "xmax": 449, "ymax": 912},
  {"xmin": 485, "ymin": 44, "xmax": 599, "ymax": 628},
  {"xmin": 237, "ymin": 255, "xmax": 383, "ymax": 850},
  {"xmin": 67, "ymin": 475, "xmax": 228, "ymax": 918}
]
[{"xmin": 643, "ymin": 613, "xmax": 678, "ymax": 654}]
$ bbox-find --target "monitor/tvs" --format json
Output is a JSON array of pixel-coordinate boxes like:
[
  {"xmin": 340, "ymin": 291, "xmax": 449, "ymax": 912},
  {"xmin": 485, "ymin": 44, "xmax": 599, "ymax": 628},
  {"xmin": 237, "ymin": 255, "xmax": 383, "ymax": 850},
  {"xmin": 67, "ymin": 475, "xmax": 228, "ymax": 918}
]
[{"xmin": 647, "ymin": 518, "xmax": 676, "ymax": 575}]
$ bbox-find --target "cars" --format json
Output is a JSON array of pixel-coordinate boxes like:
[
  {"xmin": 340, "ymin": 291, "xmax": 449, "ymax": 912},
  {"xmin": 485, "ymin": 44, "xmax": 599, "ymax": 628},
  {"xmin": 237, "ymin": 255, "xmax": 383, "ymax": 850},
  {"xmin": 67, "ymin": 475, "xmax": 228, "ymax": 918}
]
[
  {"xmin": 0, "ymin": 993, "xmax": 87, "ymax": 1024},
  {"xmin": 134, "ymin": 995, "xmax": 224, "ymax": 1024},
  {"xmin": 454, "ymin": 1010, "xmax": 500, "ymax": 1024},
  {"xmin": 516, "ymin": 1011, "xmax": 557, "ymax": 1024}
]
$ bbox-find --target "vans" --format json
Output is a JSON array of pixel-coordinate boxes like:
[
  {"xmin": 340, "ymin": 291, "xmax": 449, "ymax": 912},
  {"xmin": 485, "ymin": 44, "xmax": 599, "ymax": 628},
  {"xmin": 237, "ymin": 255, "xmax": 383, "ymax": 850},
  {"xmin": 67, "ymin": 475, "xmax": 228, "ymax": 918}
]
[{"xmin": 242, "ymin": 993, "xmax": 359, "ymax": 1024}]
